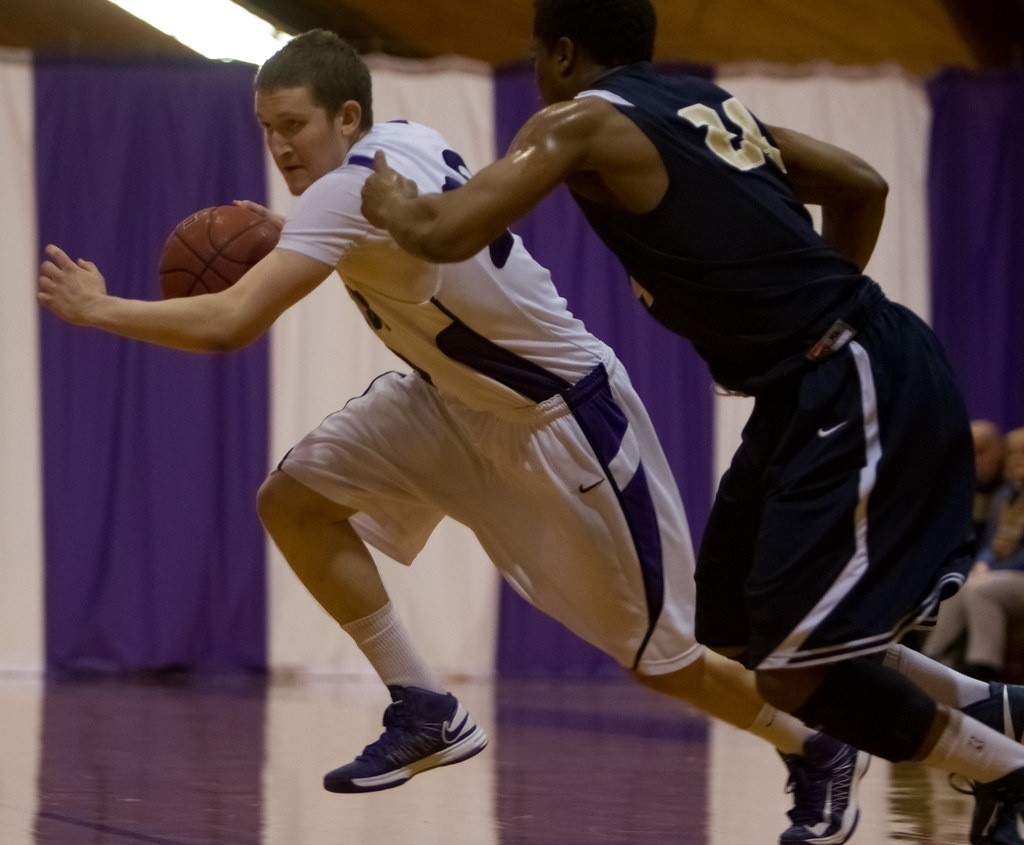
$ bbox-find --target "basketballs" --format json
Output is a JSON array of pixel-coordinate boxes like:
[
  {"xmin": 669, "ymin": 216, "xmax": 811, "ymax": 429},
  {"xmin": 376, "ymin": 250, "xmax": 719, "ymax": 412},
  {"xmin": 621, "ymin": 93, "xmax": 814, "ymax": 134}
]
[{"xmin": 157, "ymin": 203, "xmax": 281, "ymax": 301}]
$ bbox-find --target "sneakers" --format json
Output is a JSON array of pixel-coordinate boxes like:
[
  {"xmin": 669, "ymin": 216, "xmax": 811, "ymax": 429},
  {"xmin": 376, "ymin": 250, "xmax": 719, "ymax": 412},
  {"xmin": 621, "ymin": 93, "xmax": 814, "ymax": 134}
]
[
  {"xmin": 323, "ymin": 693, "xmax": 485, "ymax": 795},
  {"xmin": 779, "ymin": 727, "xmax": 871, "ymax": 845},
  {"xmin": 959, "ymin": 681, "xmax": 1024, "ymax": 742},
  {"xmin": 945, "ymin": 766, "xmax": 1024, "ymax": 843}
]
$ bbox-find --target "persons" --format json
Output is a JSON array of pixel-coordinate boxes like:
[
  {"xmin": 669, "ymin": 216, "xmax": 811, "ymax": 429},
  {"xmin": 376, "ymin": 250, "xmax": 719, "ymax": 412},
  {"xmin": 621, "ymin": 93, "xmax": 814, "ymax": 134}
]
[
  {"xmin": 358, "ymin": 0, "xmax": 1023, "ymax": 843},
  {"xmin": 921, "ymin": 414, "xmax": 1019, "ymax": 675},
  {"xmin": 40, "ymin": 32, "xmax": 870, "ymax": 841}
]
